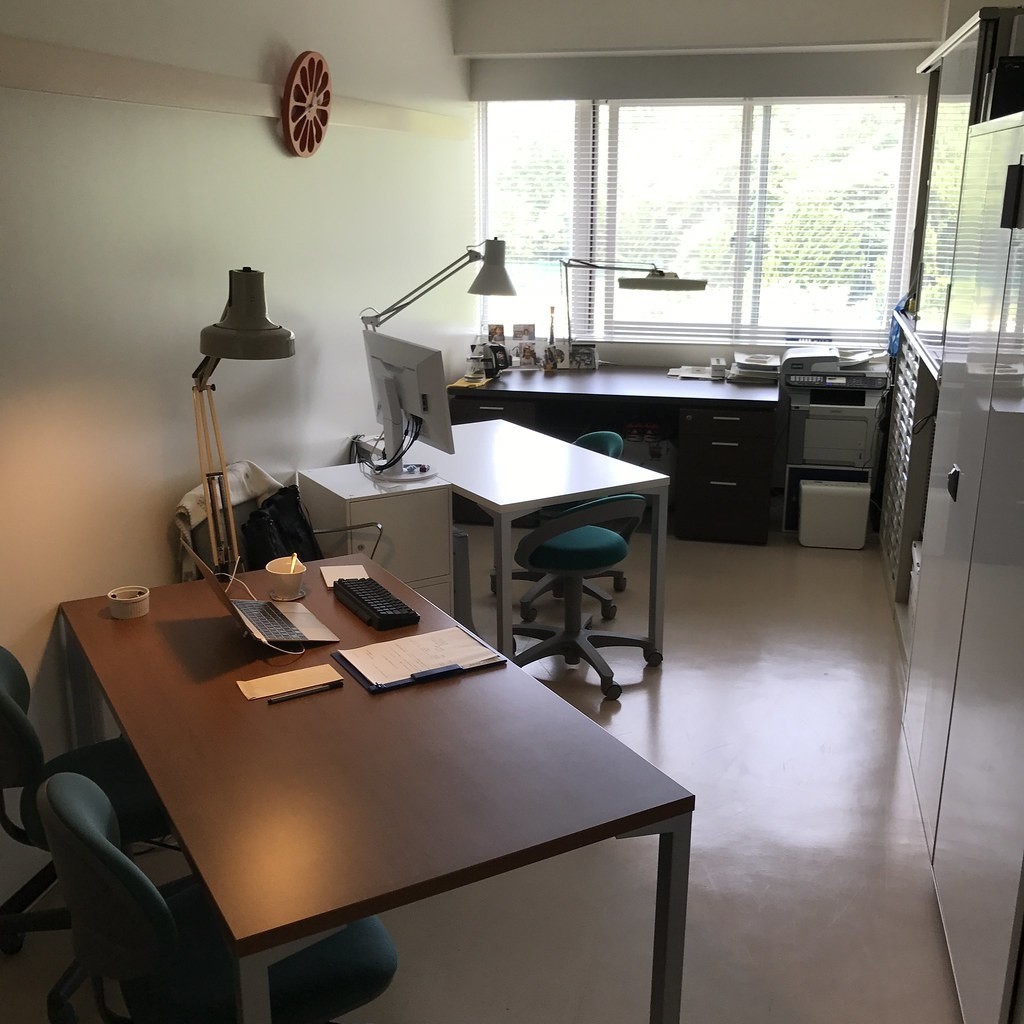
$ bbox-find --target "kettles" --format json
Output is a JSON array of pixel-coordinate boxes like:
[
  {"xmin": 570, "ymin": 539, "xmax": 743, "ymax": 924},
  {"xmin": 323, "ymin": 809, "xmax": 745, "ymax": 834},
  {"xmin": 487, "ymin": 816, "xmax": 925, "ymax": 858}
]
[{"xmin": 470, "ymin": 341, "xmax": 510, "ymax": 377}]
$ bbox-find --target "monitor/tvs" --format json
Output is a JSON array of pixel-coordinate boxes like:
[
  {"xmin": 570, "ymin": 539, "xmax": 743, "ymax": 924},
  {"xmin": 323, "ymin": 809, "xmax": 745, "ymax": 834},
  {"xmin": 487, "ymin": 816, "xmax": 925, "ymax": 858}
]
[{"xmin": 363, "ymin": 329, "xmax": 456, "ymax": 482}]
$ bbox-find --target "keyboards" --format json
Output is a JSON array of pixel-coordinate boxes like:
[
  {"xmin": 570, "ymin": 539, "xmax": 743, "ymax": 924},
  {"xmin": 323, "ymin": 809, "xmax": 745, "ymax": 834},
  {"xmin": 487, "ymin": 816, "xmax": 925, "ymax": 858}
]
[{"xmin": 334, "ymin": 577, "xmax": 420, "ymax": 630}]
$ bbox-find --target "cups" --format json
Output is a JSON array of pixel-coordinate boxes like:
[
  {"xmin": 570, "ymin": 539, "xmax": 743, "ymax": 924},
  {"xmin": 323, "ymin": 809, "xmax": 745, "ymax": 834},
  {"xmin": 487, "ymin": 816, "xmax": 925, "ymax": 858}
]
[
  {"xmin": 107, "ymin": 586, "xmax": 150, "ymax": 619},
  {"xmin": 266, "ymin": 556, "xmax": 306, "ymax": 599}
]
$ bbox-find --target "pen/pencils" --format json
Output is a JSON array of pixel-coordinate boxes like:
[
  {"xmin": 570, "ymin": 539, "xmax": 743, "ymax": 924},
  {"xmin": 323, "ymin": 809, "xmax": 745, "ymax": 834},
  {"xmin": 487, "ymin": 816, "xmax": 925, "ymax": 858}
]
[{"xmin": 267, "ymin": 681, "xmax": 344, "ymax": 703}]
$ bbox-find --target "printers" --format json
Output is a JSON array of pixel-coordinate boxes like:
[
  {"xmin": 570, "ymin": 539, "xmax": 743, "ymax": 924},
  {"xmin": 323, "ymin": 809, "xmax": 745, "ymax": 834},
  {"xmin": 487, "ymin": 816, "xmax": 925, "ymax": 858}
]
[{"xmin": 781, "ymin": 347, "xmax": 890, "ymax": 468}]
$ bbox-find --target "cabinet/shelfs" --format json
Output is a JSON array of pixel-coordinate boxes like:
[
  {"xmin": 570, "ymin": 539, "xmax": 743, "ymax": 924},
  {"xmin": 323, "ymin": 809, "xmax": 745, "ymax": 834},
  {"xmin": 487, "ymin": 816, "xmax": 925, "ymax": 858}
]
[
  {"xmin": 877, "ymin": 4, "xmax": 1024, "ymax": 1023},
  {"xmin": 449, "ymin": 398, "xmax": 536, "ymax": 529},
  {"xmin": 671, "ymin": 407, "xmax": 773, "ymax": 546},
  {"xmin": 298, "ymin": 460, "xmax": 455, "ymax": 620}
]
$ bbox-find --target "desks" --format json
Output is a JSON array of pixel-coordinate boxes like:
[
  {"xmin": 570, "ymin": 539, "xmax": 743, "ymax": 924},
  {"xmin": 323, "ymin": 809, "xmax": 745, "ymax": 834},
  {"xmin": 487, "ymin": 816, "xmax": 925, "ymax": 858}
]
[
  {"xmin": 354, "ymin": 419, "xmax": 670, "ymax": 661},
  {"xmin": 58, "ymin": 550, "xmax": 695, "ymax": 1023},
  {"xmin": 449, "ymin": 364, "xmax": 781, "ymax": 546}
]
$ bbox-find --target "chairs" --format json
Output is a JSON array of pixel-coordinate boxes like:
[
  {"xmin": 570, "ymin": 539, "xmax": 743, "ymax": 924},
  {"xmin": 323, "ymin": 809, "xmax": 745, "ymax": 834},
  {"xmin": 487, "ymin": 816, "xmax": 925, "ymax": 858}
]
[
  {"xmin": 34, "ymin": 773, "xmax": 398, "ymax": 1024},
  {"xmin": 0, "ymin": 644, "xmax": 188, "ymax": 952},
  {"xmin": 489, "ymin": 431, "xmax": 628, "ymax": 623},
  {"xmin": 512, "ymin": 493, "xmax": 664, "ymax": 701}
]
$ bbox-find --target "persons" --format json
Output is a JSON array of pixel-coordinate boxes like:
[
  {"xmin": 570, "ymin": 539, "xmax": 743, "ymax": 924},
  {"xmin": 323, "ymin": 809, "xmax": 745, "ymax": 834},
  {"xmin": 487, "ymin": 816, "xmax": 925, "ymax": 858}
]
[
  {"xmin": 521, "ymin": 329, "xmax": 529, "ymax": 340},
  {"xmin": 522, "ymin": 348, "xmax": 534, "ymax": 365},
  {"xmin": 494, "ymin": 327, "xmax": 504, "ymax": 343}
]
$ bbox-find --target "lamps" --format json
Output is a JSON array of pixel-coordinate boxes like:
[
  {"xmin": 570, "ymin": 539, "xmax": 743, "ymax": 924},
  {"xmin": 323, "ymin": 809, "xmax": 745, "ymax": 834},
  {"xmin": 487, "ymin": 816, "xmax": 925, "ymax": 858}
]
[
  {"xmin": 359, "ymin": 237, "xmax": 517, "ymax": 334},
  {"xmin": 190, "ymin": 266, "xmax": 296, "ymax": 583},
  {"xmin": 559, "ymin": 256, "xmax": 708, "ymax": 368}
]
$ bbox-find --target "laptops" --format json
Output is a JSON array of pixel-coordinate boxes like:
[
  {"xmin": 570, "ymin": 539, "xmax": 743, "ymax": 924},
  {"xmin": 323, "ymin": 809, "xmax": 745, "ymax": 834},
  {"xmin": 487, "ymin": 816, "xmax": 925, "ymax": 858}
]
[{"xmin": 180, "ymin": 538, "xmax": 340, "ymax": 642}]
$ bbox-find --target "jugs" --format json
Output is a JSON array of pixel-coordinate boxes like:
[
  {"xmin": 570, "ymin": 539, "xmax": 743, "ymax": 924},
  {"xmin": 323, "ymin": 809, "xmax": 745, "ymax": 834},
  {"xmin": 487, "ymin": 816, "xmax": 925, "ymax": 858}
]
[{"xmin": 465, "ymin": 355, "xmax": 495, "ymax": 382}]
